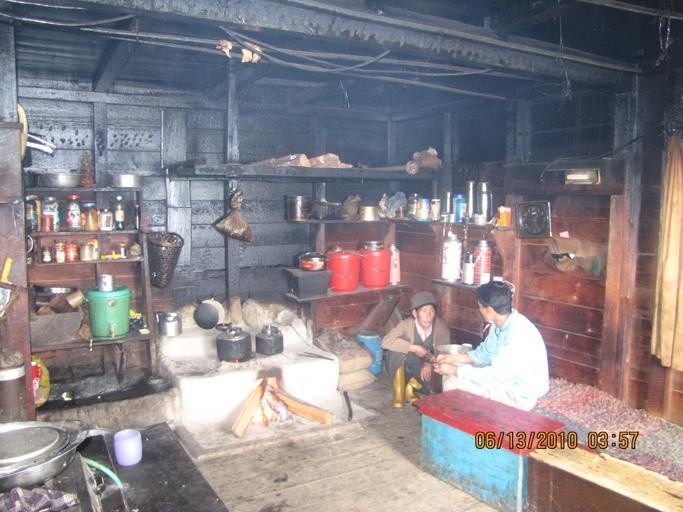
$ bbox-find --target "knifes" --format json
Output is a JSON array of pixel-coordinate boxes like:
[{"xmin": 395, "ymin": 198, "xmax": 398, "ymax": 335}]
[{"xmin": 0, "ymin": 257, "xmax": 16, "ymax": 312}]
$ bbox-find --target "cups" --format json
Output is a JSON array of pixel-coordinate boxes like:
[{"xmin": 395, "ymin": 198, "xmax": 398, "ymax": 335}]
[
  {"xmin": 480, "ymin": 273, "xmax": 490, "ymax": 286},
  {"xmin": 32, "ymin": 361, "xmax": 42, "ymax": 397},
  {"xmin": 113, "ymin": 429, "xmax": 142, "ymax": 467},
  {"xmin": 80, "ymin": 244, "xmax": 94, "ymax": 261},
  {"xmin": 96, "ymin": 273, "xmax": 114, "ymax": 292},
  {"xmin": 499, "ymin": 207, "xmax": 511, "ymax": 227}
]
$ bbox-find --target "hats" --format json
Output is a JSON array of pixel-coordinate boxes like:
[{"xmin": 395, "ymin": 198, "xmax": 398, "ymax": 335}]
[{"xmin": 409, "ymin": 291, "xmax": 438, "ymax": 310}]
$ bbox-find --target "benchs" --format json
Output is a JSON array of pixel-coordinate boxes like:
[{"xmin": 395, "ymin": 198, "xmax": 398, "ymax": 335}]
[
  {"xmin": 526, "ymin": 440, "xmax": 683, "ymax": 512},
  {"xmin": 412, "ymin": 388, "xmax": 567, "ymax": 512}
]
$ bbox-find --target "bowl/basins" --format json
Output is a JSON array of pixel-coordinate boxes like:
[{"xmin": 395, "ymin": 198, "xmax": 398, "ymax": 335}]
[
  {"xmin": 33, "ymin": 284, "xmax": 76, "ymax": 294},
  {"xmin": 148, "ymin": 378, "xmax": 170, "ymax": 391},
  {"xmin": 23, "ymin": 173, "xmax": 83, "ymax": 188},
  {"xmin": 0, "ymin": 419, "xmax": 89, "ymax": 487}
]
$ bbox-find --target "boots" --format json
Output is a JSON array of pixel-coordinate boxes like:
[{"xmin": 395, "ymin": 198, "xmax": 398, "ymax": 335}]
[
  {"xmin": 405, "ymin": 377, "xmax": 422, "ymax": 404},
  {"xmin": 392, "ymin": 362, "xmax": 407, "ymax": 409}
]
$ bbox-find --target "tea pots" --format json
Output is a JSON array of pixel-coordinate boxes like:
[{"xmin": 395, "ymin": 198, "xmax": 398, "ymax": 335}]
[{"xmin": 155, "ymin": 311, "xmax": 181, "ymax": 337}]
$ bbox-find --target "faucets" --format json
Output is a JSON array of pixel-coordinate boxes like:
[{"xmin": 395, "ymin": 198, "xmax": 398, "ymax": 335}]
[{"xmin": 109, "ymin": 323, "xmax": 116, "ymax": 338}]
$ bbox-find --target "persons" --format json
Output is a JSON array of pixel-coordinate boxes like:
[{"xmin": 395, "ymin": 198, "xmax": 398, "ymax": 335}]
[
  {"xmin": 380, "ymin": 292, "xmax": 451, "ymax": 408},
  {"xmin": 434, "ymin": 282, "xmax": 548, "ymax": 410}
]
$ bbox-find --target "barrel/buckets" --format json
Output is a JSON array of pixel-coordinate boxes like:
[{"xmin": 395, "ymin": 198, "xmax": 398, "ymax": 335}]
[
  {"xmin": 31, "ymin": 354, "xmax": 51, "ymax": 409},
  {"xmin": 286, "ymin": 194, "xmax": 313, "ymax": 222},
  {"xmin": 359, "ymin": 249, "xmax": 390, "ymax": 288},
  {"xmin": 327, "ymin": 250, "xmax": 360, "ymax": 292},
  {"xmin": 86, "ymin": 284, "xmax": 132, "ymax": 341}
]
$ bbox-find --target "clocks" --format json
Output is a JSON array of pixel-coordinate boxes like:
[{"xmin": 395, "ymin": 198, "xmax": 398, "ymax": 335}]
[{"xmin": 513, "ymin": 200, "xmax": 553, "ymax": 239}]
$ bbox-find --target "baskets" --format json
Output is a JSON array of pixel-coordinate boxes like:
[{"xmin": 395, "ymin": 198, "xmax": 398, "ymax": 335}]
[{"xmin": 147, "ymin": 230, "xmax": 185, "ymax": 288}]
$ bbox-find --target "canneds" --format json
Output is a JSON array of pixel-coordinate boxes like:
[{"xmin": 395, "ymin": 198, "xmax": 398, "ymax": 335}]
[
  {"xmin": 63, "ymin": 194, "xmax": 82, "ymax": 231},
  {"xmin": 41, "ymin": 211, "xmax": 54, "ymax": 232},
  {"xmin": 64, "ymin": 242, "xmax": 79, "ymax": 263},
  {"xmin": 53, "ymin": 239, "xmax": 66, "ymax": 265},
  {"xmin": 41, "ymin": 245, "xmax": 52, "ymax": 263},
  {"xmin": 81, "ymin": 202, "xmax": 99, "ymax": 232},
  {"xmin": 78, "ymin": 243, "xmax": 94, "ymax": 262},
  {"xmin": 99, "ymin": 207, "xmax": 114, "ymax": 232}
]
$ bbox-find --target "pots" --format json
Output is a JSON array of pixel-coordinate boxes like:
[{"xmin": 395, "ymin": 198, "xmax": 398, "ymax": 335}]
[
  {"xmin": 216, "ymin": 327, "xmax": 250, "ymax": 362},
  {"xmin": 254, "ymin": 325, "xmax": 283, "ymax": 354},
  {"xmin": 298, "ymin": 251, "xmax": 326, "ymax": 271},
  {"xmin": 194, "ymin": 298, "xmax": 218, "ymax": 329}
]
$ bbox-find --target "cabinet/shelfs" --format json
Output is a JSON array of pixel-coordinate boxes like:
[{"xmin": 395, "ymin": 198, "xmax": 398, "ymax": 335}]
[{"xmin": 24, "ymin": 186, "xmax": 161, "ymax": 386}]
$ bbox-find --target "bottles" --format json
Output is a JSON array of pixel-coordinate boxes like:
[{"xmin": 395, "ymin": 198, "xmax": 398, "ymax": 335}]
[
  {"xmin": 40, "ymin": 239, "xmax": 78, "ymax": 262},
  {"xmin": 25, "ymin": 194, "xmax": 61, "ymax": 234},
  {"xmin": 389, "ymin": 243, "xmax": 401, "ymax": 284},
  {"xmin": 133, "ymin": 204, "xmax": 140, "ymax": 230},
  {"xmin": 463, "ymin": 252, "xmax": 473, "ymax": 285},
  {"xmin": 443, "ymin": 180, "xmax": 492, "ymax": 222},
  {"xmin": 113, "ymin": 194, "xmax": 125, "ymax": 233}
]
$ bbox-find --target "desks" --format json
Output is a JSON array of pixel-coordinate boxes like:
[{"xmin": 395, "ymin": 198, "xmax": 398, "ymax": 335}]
[{"xmin": 0, "ymin": 422, "xmax": 228, "ymax": 512}]
